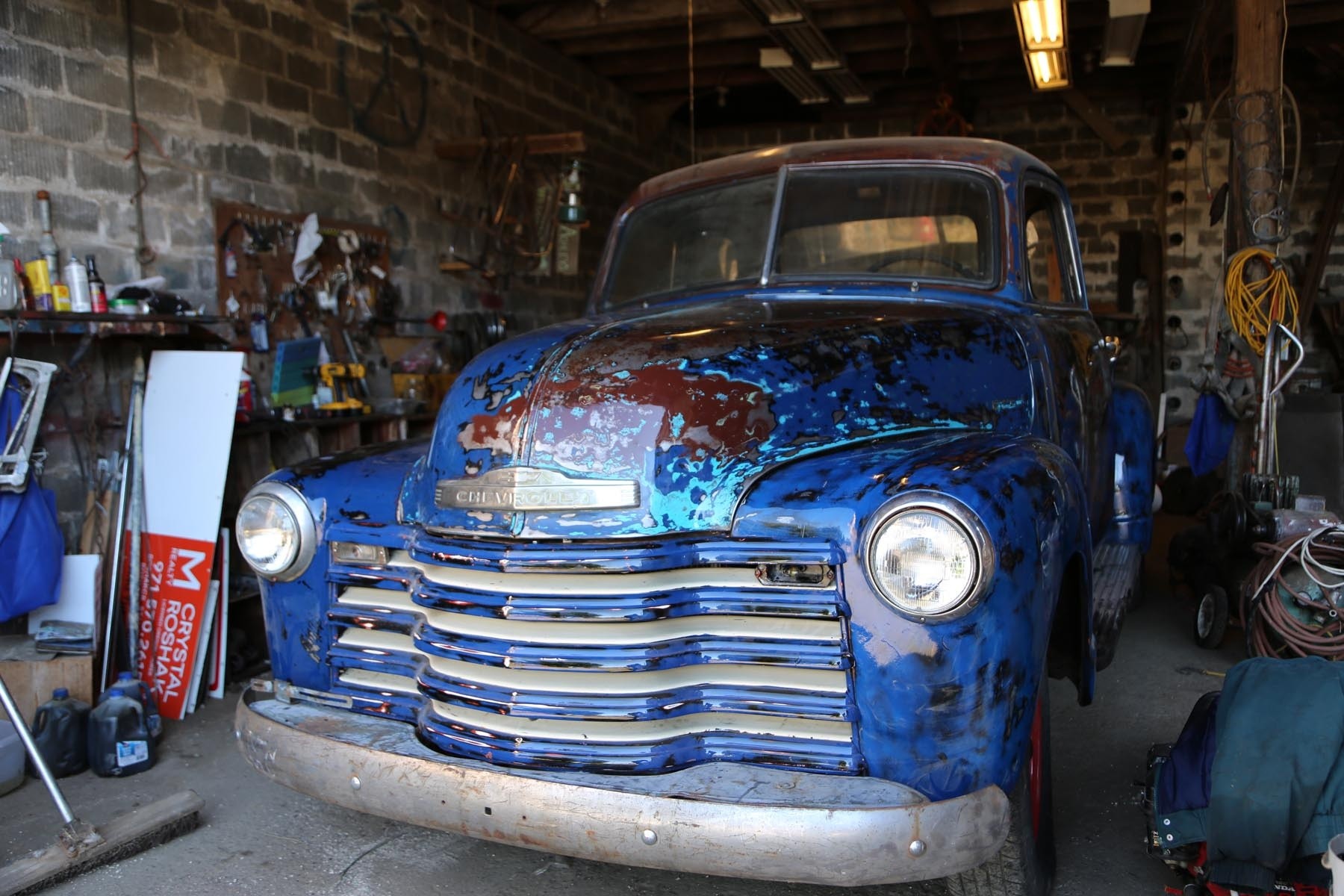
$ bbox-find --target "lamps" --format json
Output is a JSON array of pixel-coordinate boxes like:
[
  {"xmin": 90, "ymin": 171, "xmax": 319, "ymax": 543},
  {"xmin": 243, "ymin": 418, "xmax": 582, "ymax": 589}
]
[{"xmin": 1009, "ymin": 0, "xmax": 1075, "ymax": 94}]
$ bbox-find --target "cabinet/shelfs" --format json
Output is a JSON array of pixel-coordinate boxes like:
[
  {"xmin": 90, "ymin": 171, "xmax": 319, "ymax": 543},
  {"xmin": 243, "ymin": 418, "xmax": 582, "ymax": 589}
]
[{"xmin": 440, "ymin": 133, "xmax": 678, "ymax": 293}]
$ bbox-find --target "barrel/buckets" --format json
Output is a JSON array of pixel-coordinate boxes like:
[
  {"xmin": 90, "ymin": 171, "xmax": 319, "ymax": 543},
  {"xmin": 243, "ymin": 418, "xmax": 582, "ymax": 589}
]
[{"xmin": 26, "ymin": 672, "xmax": 163, "ymax": 780}]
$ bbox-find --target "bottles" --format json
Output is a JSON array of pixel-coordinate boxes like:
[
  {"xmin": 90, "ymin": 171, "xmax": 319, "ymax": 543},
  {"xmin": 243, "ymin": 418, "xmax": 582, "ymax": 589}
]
[{"xmin": 22, "ymin": 254, "xmax": 107, "ymax": 313}]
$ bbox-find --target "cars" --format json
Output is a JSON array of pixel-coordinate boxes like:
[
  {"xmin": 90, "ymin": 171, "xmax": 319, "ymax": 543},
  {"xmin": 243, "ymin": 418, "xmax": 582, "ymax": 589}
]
[{"xmin": 234, "ymin": 139, "xmax": 1159, "ymax": 896}]
